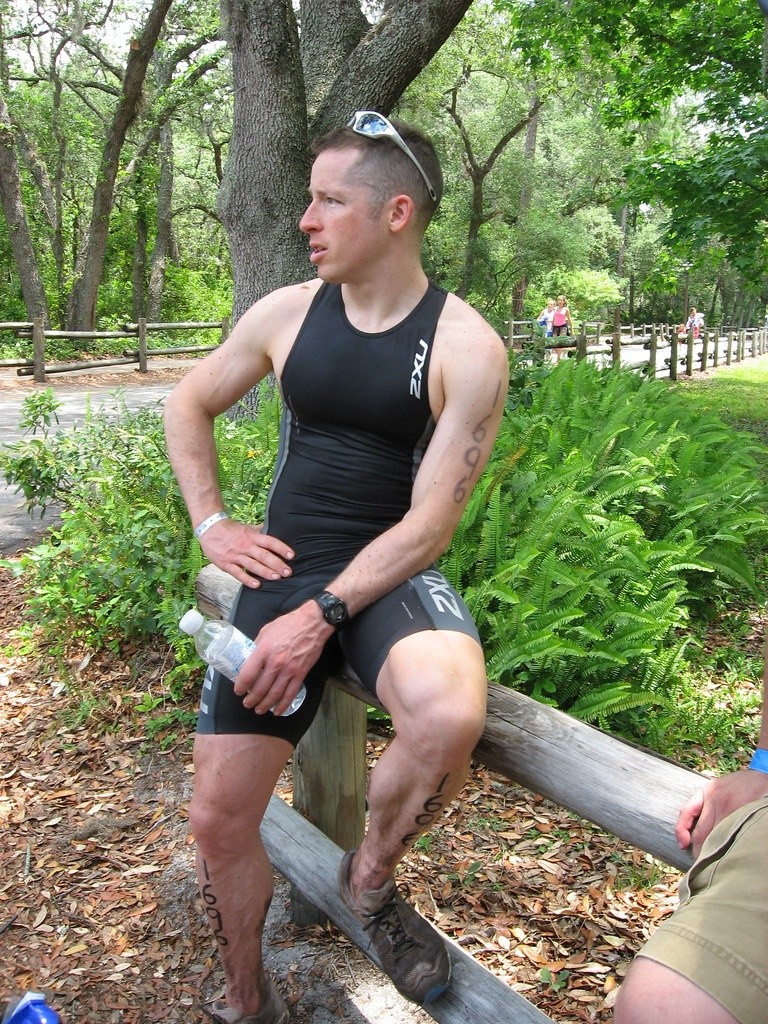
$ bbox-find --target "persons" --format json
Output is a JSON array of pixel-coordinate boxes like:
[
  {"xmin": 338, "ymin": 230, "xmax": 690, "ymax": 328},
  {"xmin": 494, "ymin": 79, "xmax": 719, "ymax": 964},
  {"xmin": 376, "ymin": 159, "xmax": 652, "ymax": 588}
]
[
  {"xmin": 612, "ymin": 646, "xmax": 768, "ymax": 1024},
  {"xmin": 677, "ymin": 307, "xmax": 704, "ymax": 347},
  {"xmin": 161, "ymin": 110, "xmax": 510, "ymax": 1024},
  {"xmin": 551, "ymin": 295, "xmax": 574, "ymax": 365},
  {"xmin": 537, "ymin": 300, "xmax": 556, "ymax": 365}
]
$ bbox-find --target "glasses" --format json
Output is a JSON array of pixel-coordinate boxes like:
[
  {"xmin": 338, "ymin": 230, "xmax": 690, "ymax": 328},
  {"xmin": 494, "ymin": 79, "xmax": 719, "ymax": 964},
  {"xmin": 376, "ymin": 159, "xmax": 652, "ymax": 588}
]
[{"xmin": 344, "ymin": 109, "xmax": 437, "ymax": 201}]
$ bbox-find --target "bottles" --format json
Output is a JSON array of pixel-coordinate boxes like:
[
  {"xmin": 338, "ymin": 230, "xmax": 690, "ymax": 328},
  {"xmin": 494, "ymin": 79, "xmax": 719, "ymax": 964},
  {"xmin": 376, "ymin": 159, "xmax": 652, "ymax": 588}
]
[{"xmin": 178, "ymin": 610, "xmax": 306, "ymax": 718}]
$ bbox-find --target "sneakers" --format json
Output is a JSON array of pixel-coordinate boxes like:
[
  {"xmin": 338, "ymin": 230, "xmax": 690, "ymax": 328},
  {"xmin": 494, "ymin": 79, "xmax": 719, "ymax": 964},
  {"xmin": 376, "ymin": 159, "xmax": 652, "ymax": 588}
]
[
  {"xmin": 200, "ymin": 973, "xmax": 290, "ymax": 1024},
  {"xmin": 339, "ymin": 850, "xmax": 451, "ymax": 1004}
]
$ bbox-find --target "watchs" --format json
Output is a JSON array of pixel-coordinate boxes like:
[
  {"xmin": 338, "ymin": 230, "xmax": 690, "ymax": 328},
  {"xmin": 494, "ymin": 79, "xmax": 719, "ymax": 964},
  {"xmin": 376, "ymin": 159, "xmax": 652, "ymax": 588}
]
[{"xmin": 313, "ymin": 591, "xmax": 350, "ymax": 630}]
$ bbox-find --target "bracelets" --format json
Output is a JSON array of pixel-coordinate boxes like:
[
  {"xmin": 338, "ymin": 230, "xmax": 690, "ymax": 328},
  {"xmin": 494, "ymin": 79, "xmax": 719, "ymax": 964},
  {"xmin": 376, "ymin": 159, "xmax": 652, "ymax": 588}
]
[
  {"xmin": 748, "ymin": 748, "xmax": 768, "ymax": 775},
  {"xmin": 194, "ymin": 512, "xmax": 229, "ymax": 540}
]
